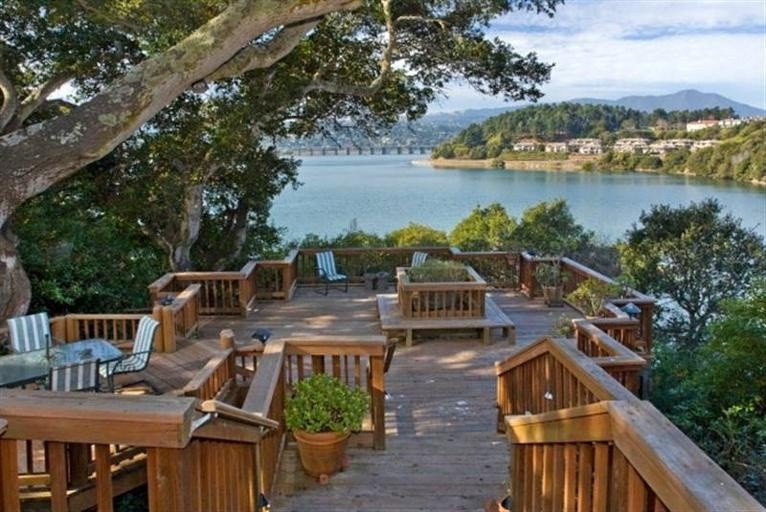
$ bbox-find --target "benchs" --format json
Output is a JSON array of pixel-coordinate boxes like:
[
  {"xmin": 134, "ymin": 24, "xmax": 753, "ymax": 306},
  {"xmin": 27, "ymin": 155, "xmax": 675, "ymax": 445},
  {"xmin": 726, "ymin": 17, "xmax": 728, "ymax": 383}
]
[{"xmin": 374, "ymin": 292, "xmax": 517, "ymax": 346}]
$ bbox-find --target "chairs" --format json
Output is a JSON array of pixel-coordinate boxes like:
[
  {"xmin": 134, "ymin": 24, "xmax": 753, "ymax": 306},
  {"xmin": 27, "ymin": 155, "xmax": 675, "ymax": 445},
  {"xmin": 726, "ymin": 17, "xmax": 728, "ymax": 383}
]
[
  {"xmin": 410, "ymin": 251, "xmax": 429, "ymax": 266},
  {"xmin": 312, "ymin": 249, "xmax": 348, "ymax": 296},
  {"xmin": 0, "ymin": 310, "xmax": 161, "ymax": 396}
]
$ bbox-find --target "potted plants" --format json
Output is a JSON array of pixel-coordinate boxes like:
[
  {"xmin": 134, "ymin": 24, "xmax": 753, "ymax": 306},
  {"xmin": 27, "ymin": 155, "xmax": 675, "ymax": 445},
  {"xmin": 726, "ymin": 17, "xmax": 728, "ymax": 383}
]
[
  {"xmin": 282, "ymin": 370, "xmax": 372, "ymax": 478},
  {"xmin": 535, "ymin": 260, "xmax": 572, "ymax": 309},
  {"xmin": 407, "ymin": 257, "xmax": 470, "ymax": 310}
]
